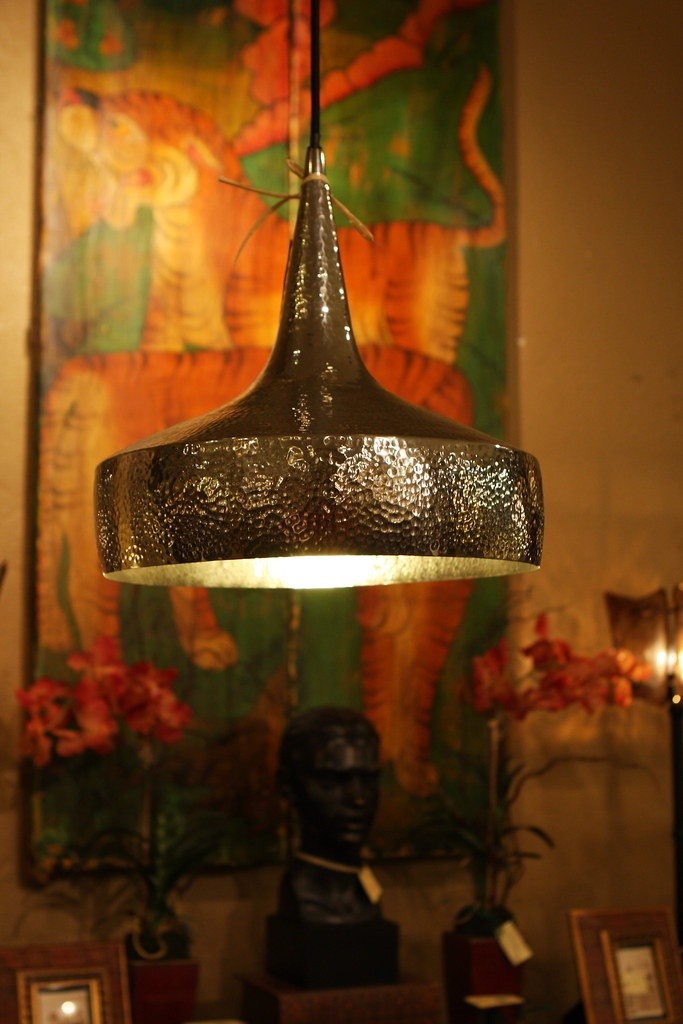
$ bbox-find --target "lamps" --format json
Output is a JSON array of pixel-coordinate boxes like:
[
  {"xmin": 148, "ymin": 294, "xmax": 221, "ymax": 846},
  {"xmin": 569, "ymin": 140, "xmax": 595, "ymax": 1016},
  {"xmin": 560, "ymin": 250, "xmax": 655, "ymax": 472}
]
[
  {"xmin": 92, "ymin": 0, "xmax": 546, "ymax": 592},
  {"xmin": 602, "ymin": 583, "xmax": 683, "ymax": 951}
]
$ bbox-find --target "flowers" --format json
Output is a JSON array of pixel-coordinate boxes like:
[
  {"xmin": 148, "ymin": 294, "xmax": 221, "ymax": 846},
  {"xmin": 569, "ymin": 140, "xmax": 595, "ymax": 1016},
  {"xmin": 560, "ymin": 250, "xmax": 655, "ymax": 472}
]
[
  {"xmin": 17, "ymin": 649, "xmax": 234, "ymax": 960},
  {"xmin": 405, "ymin": 615, "xmax": 633, "ymax": 935}
]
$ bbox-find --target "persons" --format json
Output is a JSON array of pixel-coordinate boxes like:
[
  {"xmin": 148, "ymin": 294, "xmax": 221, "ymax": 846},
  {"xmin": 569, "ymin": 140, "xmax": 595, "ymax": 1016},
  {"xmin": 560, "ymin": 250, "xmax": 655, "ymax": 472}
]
[{"xmin": 275, "ymin": 705, "xmax": 384, "ymax": 924}]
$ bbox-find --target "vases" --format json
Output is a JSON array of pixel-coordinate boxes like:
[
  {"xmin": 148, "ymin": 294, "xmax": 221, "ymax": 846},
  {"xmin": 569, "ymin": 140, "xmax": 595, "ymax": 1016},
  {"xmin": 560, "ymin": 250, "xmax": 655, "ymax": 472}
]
[
  {"xmin": 436, "ymin": 931, "xmax": 527, "ymax": 1018},
  {"xmin": 121, "ymin": 928, "xmax": 203, "ymax": 1024}
]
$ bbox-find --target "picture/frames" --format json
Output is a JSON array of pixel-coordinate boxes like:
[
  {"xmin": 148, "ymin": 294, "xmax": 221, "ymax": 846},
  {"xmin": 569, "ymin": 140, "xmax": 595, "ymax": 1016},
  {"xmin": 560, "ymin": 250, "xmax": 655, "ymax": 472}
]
[
  {"xmin": 566, "ymin": 906, "xmax": 683, "ymax": 1024},
  {"xmin": 0, "ymin": 935, "xmax": 133, "ymax": 1024}
]
